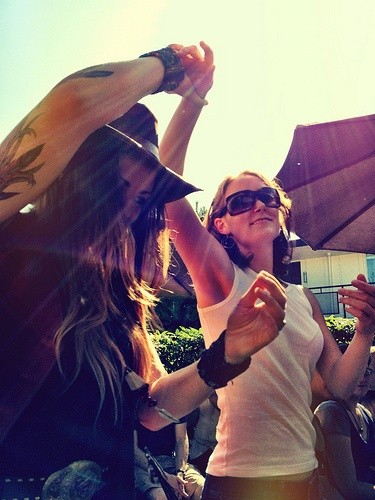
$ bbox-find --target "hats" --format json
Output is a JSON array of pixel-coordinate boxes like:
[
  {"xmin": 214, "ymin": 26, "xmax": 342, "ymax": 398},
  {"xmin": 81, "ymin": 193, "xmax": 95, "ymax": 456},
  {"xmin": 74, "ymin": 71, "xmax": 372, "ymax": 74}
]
[{"xmin": 104, "ymin": 103, "xmax": 203, "ymax": 205}]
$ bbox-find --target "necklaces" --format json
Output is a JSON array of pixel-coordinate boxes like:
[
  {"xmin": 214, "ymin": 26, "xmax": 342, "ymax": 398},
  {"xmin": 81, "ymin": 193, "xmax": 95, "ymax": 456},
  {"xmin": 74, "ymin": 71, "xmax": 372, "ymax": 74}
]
[{"xmin": 344, "ymin": 402, "xmax": 365, "ymax": 432}]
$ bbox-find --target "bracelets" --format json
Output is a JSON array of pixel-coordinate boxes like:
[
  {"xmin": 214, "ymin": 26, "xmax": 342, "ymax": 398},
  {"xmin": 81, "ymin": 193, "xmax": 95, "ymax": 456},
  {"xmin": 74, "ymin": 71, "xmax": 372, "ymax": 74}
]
[
  {"xmin": 175, "ymin": 469, "xmax": 186, "ymax": 480},
  {"xmin": 140, "ymin": 47, "xmax": 185, "ymax": 95},
  {"xmin": 198, "ymin": 330, "xmax": 251, "ymax": 390}
]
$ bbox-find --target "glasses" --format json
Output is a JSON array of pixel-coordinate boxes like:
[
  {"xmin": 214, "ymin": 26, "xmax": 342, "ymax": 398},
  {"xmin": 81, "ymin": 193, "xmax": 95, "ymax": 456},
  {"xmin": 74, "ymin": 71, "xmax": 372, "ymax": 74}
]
[
  {"xmin": 364, "ymin": 368, "xmax": 374, "ymax": 375},
  {"xmin": 219, "ymin": 187, "xmax": 280, "ymax": 219}
]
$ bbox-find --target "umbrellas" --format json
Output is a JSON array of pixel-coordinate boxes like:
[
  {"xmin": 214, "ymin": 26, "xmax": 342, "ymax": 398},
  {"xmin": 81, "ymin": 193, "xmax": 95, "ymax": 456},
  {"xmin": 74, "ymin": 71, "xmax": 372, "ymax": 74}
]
[{"xmin": 269, "ymin": 113, "xmax": 375, "ymax": 254}]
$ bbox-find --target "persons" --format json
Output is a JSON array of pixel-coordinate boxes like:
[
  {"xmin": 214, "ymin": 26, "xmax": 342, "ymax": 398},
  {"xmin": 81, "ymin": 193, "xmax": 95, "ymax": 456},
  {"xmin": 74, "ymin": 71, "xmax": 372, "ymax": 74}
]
[
  {"xmin": 0, "ymin": 43, "xmax": 287, "ymax": 500},
  {"xmin": 189, "ymin": 391, "xmax": 219, "ymax": 478},
  {"xmin": 311, "ymin": 366, "xmax": 347, "ymax": 500},
  {"xmin": 156, "ymin": 40, "xmax": 375, "ymax": 500},
  {"xmin": 312, "ymin": 343, "xmax": 375, "ymax": 500},
  {"xmin": 134, "ymin": 359, "xmax": 205, "ymax": 500},
  {"xmin": 355, "ymin": 346, "xmax": 374, "ymax": 421}
]
